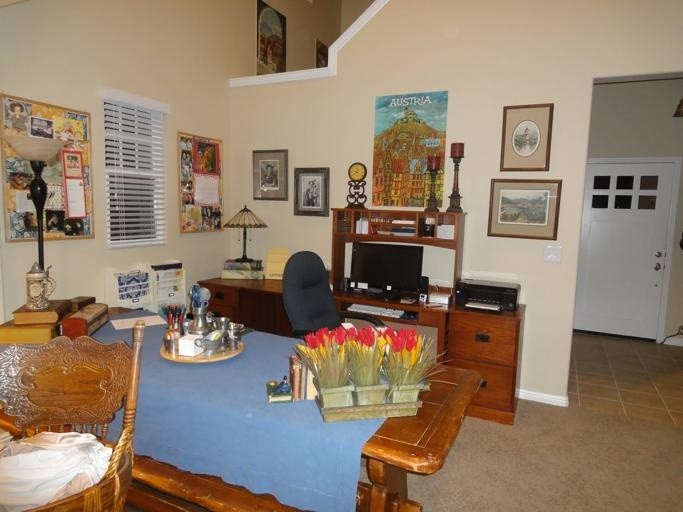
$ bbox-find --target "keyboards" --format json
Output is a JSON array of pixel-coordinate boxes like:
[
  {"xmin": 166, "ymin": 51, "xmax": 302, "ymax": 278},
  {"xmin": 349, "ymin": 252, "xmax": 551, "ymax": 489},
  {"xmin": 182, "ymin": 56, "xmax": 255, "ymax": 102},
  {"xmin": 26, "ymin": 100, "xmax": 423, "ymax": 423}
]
[{"xmin": 347, "ymin": 304, "xmax": 405, "ymax": 319}]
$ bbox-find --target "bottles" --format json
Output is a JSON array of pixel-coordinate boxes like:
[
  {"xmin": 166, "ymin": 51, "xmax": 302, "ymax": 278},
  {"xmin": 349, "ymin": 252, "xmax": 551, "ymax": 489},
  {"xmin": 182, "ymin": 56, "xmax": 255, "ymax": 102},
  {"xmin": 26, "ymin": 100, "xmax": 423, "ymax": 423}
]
[{"xmin": 24, "ymin": 263, "xmax": 56, "ymax": 311}]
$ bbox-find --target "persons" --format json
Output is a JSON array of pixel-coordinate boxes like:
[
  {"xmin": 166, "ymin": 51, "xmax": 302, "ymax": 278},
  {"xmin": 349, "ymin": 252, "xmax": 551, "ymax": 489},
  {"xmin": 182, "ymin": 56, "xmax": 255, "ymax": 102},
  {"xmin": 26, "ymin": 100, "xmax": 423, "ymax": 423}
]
[
  {"xmin": 202, "ymin": 207, "xmax": 221, "ymax": 230},
  {"xmin": 186, "ymin": 139, "xmax": 193, "ymax": 149},
  {"xmin": 180, "ymin": 137, "xmax": 188, "ymax": 148},
  {"xmin": 262, "ymin": 165, "xmax": 277, "ymax": 187},
  {"xmin": 183, "ymin": 181, "xmax": 193, "ymax": 203},
  {"xmin": 182, "ymin": 152, "xmax": 188, "ymax": 169},
  {"xmin": 186, "ymin": 151, "xmax": 192, "ymax": 167}
]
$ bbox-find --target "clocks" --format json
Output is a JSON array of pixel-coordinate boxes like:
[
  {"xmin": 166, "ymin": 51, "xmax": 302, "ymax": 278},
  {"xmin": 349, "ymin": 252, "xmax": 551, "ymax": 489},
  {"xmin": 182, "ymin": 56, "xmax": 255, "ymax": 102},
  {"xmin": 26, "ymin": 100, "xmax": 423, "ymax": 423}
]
[{"xmin": 349, "ymin": 162, "xmax": 367, "ymax": 182}]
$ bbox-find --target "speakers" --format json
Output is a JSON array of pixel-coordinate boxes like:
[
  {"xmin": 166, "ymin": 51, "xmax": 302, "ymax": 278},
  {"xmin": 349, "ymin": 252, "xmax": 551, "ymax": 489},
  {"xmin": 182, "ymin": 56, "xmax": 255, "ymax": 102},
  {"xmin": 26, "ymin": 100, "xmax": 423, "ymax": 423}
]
[
  {"xmin": 421, "ymin": 276, "xmax": 429, "ymax": 303},
  {"xmin": 340, "ymin": 278, "xmax": 349, "ymax": 292}
]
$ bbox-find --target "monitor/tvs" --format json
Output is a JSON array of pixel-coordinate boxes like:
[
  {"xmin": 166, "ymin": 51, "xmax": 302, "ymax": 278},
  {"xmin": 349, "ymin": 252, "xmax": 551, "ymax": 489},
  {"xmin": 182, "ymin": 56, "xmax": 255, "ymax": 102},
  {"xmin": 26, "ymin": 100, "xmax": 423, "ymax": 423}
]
[{"xmin": 350, "ymin": 243, "xmax": 423, "ymax": 302}]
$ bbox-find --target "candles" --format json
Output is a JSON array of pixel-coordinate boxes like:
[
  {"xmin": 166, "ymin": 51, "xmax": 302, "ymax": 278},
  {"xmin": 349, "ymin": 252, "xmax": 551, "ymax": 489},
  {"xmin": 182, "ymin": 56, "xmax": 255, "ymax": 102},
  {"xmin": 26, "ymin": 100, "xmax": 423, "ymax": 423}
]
[{"xmin": 427, "ymin": 143, "xmax": 465, "ymax": 169}]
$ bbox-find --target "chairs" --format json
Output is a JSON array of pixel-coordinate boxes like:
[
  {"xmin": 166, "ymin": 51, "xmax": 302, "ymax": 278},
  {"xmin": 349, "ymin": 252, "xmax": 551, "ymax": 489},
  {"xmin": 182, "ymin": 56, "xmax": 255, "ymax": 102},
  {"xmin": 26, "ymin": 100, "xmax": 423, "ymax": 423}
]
[{"xmin": 0, "ymin": 317, "xmax": 146, "ymax": 512}]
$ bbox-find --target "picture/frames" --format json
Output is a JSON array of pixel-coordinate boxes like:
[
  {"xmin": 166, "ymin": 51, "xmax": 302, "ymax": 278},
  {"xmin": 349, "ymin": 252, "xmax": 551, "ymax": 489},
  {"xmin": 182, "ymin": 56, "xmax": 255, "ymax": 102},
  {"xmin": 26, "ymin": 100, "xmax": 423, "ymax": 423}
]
[
  {"xmin": 252, "ymin": 149, "xmax": 330, "ymax": 217},
  {"xmin": 176, "ymin": 133, "xmax": 226, "ymax": 232},
  {"xmin": 487, "ymin": 103, "xmax": 563, "ymax": 241},
  {"xmin": 2, "ymin": 90, "xmax": 94, "ymax": 243}
]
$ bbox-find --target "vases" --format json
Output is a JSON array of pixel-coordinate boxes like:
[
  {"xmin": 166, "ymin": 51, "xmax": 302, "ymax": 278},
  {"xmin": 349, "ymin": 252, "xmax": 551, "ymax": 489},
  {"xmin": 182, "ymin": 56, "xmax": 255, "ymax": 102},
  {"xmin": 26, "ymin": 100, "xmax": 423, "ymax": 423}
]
[{"xmin": 312, "ymin": 376, "xmax": 423, "ymax": 408}]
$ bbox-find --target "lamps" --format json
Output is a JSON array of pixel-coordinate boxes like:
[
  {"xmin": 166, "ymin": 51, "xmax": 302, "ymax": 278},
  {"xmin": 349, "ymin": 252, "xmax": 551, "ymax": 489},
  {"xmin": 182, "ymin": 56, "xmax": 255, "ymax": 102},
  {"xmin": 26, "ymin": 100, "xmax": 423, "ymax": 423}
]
[
  {"xmin": 222, "ymin": 203, "xmax": 269, "ymax": 261},
  {"xmin": 7, "ymin": 133, "xmax": 63, "ymax": 292}
]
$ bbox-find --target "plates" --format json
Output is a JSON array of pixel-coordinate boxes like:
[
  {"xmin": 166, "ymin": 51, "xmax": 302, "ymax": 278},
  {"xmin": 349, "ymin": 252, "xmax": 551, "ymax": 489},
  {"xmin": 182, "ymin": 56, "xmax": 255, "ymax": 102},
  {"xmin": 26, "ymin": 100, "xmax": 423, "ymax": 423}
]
[{"xmin": 159, "ymin": 341, "xmax": 244, "ymax": 363}]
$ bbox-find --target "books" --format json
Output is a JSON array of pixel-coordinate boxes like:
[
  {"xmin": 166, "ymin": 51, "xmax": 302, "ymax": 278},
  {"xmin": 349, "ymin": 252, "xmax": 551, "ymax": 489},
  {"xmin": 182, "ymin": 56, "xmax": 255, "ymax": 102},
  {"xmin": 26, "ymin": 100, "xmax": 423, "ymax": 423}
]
[
  {"xmin": 11, "ymin": 299, "xmax": 71, "ymax": 325},
  {"xmin": 289, "ymin": 355, "xmax": 317, "ymax": 401}
]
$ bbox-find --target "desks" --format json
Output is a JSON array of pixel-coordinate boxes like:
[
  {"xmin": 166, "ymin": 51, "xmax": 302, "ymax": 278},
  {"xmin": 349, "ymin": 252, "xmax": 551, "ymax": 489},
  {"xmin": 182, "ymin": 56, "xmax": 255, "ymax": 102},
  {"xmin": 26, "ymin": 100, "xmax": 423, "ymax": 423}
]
[
  {"xmin": 329, "ymin": 205, "xmax": 468, "ymax": 295},
  {"xmin": 38, "ymin": 298, "xmax": 484, "ymax": 512},
  {"xmin": 196, "ymin": 271, "xmax": 528, "ymax": 426}
]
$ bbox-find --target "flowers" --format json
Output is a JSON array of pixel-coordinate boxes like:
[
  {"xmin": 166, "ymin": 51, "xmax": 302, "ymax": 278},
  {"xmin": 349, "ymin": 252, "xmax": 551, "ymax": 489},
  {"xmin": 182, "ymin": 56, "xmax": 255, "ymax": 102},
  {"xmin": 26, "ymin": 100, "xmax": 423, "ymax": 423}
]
[{"xmin": 293, "ymin": 323, "xmax": 453, "ymax": 388}]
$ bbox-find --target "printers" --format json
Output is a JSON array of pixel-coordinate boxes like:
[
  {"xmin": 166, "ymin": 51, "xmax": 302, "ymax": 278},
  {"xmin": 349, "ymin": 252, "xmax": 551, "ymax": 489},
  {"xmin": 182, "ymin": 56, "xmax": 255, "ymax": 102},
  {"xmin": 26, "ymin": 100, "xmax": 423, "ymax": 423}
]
[{"xmin": 453, "ymin": 279, "xmax": 522, "ymax": 314}]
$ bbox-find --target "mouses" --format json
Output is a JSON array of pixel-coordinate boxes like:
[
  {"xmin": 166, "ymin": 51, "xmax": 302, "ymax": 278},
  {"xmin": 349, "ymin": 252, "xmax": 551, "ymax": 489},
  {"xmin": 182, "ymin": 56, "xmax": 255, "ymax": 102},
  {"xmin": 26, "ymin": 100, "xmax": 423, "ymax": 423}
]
[{"xmin": 407, "ymin": 312, "xmax": 416, "ymax": 319}]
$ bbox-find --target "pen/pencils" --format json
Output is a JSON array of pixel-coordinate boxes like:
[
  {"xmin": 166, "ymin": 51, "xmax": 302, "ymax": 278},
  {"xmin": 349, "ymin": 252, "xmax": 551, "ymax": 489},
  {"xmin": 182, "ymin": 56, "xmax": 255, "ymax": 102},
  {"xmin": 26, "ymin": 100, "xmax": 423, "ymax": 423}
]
[
  {"xmin": 163, "ymin": 304, "xmax": 187, "ymax": 325},
  {"xmin": 426, "ymin": 306, "xmax": 444, "ymax": 307}
]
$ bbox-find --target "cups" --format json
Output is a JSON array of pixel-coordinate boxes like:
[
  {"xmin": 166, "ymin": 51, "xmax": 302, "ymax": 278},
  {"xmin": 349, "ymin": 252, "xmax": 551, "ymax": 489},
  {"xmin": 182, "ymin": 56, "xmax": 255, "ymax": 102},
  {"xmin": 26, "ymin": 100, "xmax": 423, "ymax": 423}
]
[{"xmin": 162, "ymin": 299, "xmax": 242, "ymax": 360}]
